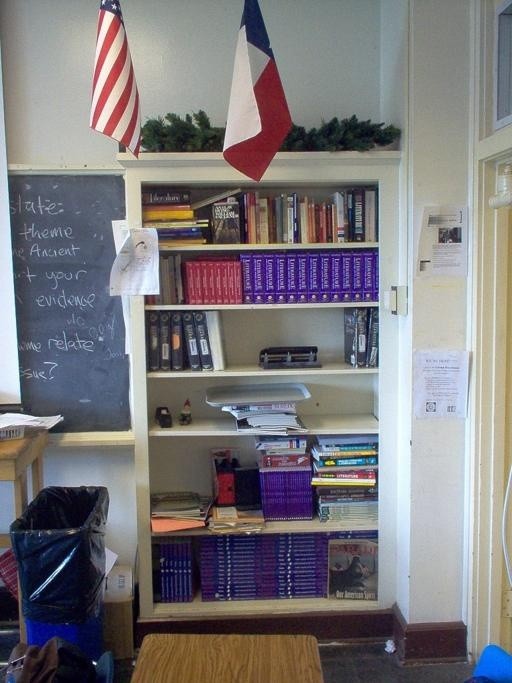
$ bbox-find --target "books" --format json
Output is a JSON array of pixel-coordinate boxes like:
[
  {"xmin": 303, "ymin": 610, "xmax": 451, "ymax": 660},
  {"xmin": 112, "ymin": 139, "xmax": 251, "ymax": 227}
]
[
  {"xmin": 143, "ymin": 308, "xmax": 226, "ymax": 373},
  {"xmin": 218, "ymin": 402, "xmax": 308, "ymax": 434},
  {"xmin": 139, "ymin": 183, "xmax": 378, "ymax": 306},
  {"xmin": 339, "ymin": 306, "xmax": 380, "ymax": 369},
  {"xmin": 151, "ymin": 434, "xmax": 378, "ymax": 604}
]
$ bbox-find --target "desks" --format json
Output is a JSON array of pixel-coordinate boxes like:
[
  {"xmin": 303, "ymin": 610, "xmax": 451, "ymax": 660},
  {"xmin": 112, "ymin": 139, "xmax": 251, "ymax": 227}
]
[
  {"xmin": 132, "ymin": 634, "xmax": 323, "ymax": 683},
  {"xmin": 1, "ymin": 431, "xmax": 49, "ymax": 645}
]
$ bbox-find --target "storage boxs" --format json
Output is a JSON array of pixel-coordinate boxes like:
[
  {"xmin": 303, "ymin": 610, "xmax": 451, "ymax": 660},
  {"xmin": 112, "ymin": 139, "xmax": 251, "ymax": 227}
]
[{"xmin": 99, "ymin": 568, "xmax": 133, "ymax": 661}]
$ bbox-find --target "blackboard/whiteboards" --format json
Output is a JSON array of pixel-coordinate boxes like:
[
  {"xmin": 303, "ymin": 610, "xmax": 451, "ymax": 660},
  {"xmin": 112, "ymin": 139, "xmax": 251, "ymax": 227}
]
[{"xmin": 7, "ymin": 163, "xmax": 136, "ymax": 447}]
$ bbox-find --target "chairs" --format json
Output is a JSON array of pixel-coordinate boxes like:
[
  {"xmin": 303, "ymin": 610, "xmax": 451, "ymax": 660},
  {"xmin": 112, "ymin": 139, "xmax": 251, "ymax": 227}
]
[{"xmin": 468, "ymin": 645, "xmax": 512, "ymax": 682}]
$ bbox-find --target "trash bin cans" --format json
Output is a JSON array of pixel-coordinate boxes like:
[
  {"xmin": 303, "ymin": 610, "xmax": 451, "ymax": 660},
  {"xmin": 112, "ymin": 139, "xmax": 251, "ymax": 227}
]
[{"xmin": 11, "ymin": 486, "xmax": 105, "ymax": 660}]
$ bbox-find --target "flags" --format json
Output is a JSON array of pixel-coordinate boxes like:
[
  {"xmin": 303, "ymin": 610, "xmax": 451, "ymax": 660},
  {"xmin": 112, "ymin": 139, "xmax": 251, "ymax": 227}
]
[
  {"xmin": 219, "ymin": 1, "xmax": 296, "ymax": 185},
  {"xmin": 86, "ymin": 0, "xmax": 144, "ymax": 161}
]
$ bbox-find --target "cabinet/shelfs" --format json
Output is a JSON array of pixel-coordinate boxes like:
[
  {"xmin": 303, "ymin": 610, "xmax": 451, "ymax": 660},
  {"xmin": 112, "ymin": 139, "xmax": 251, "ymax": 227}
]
[{"xmin": 120, "ymin": 148, "xmax": 397, "ymax": 616}]
